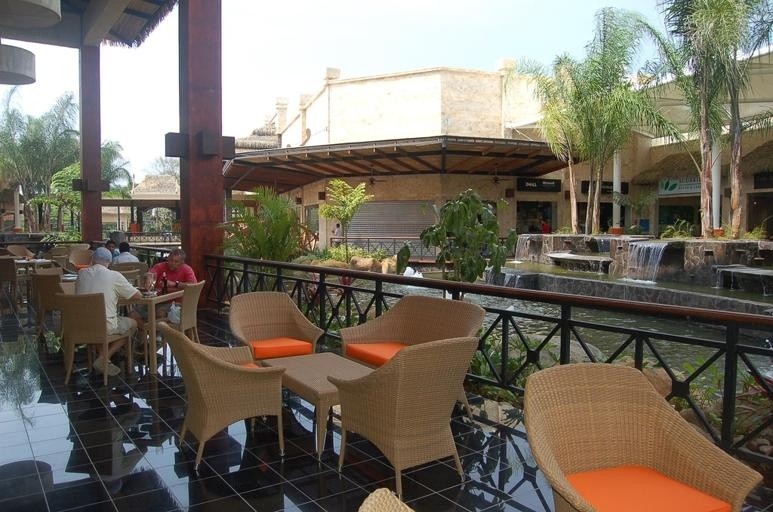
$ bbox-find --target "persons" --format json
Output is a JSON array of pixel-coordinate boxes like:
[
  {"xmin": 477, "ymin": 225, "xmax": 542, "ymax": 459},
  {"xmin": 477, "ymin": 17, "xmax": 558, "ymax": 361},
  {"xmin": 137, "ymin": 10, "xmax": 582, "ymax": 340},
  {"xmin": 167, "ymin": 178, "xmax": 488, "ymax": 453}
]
[
  {"xmin": 105, "ymin": 241, "xmax": 120, "ymax": 257},
  {"xmin": 111, "ymin": 243, "xmax": 140, "ymax": 265},
  {"xmin": 331, "ymin": 224, "xmax": 342, "ymax": 246},
  {"xmin": 74, "ymin": 246, "xmax": 141, "ymax": 376},
  {"xmin": 538, "ymin": 218, "xmax": 549, "ymax": 234},
  {"xmin": 128, "ymin": 250, "xmax": 196, "ymax": 339}
]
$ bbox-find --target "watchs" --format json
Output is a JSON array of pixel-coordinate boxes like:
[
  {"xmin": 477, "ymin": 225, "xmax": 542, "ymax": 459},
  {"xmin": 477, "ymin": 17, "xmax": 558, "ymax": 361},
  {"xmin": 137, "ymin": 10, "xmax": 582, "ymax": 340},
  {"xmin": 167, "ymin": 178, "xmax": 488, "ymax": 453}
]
[{"xmin": 174, "ymin": 281, "xmax": 180, "ymax": 287}]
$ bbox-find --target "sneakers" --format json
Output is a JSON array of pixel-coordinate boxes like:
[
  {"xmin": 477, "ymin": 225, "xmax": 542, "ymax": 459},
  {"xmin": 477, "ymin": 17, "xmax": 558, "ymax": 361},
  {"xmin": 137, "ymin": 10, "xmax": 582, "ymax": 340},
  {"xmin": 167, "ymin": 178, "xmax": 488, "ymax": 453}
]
[
  {"xmin": 92, "ymin": 357, "xmax": 120, "ymax": 377},
  {"xmin": 133, "ymin": 342, "xmax": 148, "ymax": 355}
]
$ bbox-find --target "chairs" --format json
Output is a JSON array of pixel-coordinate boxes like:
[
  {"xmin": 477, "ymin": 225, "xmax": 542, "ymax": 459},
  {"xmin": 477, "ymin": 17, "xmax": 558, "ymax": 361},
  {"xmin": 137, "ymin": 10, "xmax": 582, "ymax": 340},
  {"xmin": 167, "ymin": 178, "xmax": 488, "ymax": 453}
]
[
  {"xmin": 230, "ymin": 290, "xmax": 325, "ymax": 358},
  {"xmin": 0, "ymin": 240, "xmax": 205, "ymax": 384},
  {"xmin": 523, "ymin": 362, "xmax": 763, "ymax": 511},
  {"xmin": 339, "ymin": 296, "xmax": 485, "ymax": 414},
  {"xmin": 157, "ymin": 322, "xmax": 288, "ymax": 468},
  {"xmin": 327, "ymin": 337, "xmax": 479, "ymax": 498}
]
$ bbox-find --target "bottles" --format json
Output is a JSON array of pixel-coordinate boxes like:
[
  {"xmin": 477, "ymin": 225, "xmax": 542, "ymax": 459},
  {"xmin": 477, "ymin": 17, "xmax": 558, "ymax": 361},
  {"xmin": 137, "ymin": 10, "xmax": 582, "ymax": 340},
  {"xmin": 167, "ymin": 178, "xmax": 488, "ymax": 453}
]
[{"xmin": 160, "ymin": 276, "xmax": 167, "ymax": 293}]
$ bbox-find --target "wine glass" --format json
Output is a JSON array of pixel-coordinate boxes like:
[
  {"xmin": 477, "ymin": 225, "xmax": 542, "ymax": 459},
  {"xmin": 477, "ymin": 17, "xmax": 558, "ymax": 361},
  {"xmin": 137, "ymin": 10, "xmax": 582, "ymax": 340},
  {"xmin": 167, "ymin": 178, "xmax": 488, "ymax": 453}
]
[
  {"xmin": 149, "ymin": 273, "xmax": 156, "ymax": 296},
  {"xmin": 143, "ymin": 273, "xmax": 152, "ymax": 297}
]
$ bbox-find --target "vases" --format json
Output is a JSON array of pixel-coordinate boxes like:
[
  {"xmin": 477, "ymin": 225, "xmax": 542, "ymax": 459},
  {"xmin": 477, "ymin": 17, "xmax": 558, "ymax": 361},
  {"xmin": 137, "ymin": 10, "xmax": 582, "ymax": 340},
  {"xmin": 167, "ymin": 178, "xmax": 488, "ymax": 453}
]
[{"xmin": 13, "ymin": 227, "xmax": 22, "ymax": 234}]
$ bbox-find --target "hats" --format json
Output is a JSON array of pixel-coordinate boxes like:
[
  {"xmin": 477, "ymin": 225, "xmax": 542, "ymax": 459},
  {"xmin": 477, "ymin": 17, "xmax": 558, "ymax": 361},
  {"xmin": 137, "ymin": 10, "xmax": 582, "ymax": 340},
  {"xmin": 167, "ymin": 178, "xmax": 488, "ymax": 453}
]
[{"xmin": 90, "ymin": 246, "xmax": 112, "ymax": 263}]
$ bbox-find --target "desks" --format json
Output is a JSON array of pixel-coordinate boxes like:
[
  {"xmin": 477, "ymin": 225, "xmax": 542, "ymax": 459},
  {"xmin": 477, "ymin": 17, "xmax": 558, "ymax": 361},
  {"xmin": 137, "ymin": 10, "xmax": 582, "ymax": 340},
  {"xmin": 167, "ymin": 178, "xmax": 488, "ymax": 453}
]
[{"xmin": 262, "ymin": 351, "xmax": 374, "ymax": 453}]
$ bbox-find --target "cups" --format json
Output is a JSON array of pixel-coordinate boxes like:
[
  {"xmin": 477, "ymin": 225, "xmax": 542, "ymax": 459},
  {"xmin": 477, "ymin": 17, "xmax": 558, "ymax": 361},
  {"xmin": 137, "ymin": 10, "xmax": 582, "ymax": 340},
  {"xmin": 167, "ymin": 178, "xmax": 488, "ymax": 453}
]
[{"xmin": 134, "ymin": 279, "xmax": 139, "ymax": 289}]
